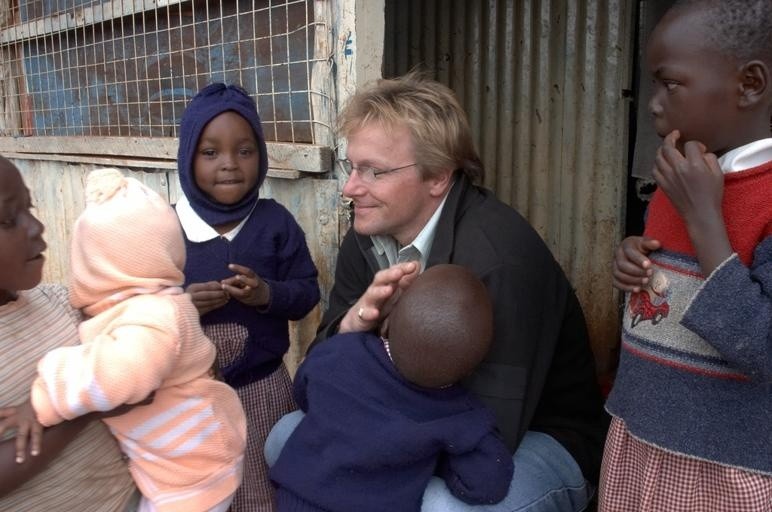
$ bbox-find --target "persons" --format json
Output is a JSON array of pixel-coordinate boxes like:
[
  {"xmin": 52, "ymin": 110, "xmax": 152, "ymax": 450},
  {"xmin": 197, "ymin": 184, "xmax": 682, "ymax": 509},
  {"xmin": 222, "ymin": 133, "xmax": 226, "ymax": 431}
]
[
  {"xmin": 596, "ymin": 3, "xmax": 772, "ymax": 512},
  {"xmin": 0, "ymin": 165, "xmax": 250, "ymax": 512},
  {"xmin": 260, "ymin": 61, "xmax": 609, "ymax": 511},
  {"xmin": 270, "ymin": 264, "xmax": 517, "ymax": 511},
  {"xmin": 1, "ymin": 155, "xmax": 141, "ymax": 512},
  {"xmin": 165, "ymin": 79, "xmax": 321, "ymax": 512}
]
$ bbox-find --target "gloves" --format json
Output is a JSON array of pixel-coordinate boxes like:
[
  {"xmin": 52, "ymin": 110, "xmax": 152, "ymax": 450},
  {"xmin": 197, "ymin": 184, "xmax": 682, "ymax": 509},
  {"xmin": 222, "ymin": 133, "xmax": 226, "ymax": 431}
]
[{"xmin": 335, "ymin": 158, "xmax": 416, "ymax": 185}]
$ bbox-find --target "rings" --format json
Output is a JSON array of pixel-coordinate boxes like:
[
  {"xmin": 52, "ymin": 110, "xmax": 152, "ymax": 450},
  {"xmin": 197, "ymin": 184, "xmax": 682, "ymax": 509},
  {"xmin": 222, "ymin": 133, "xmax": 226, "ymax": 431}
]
[{"xmin": 358, "ymin": 306, "xmax": 366, "ymax": 322}]
